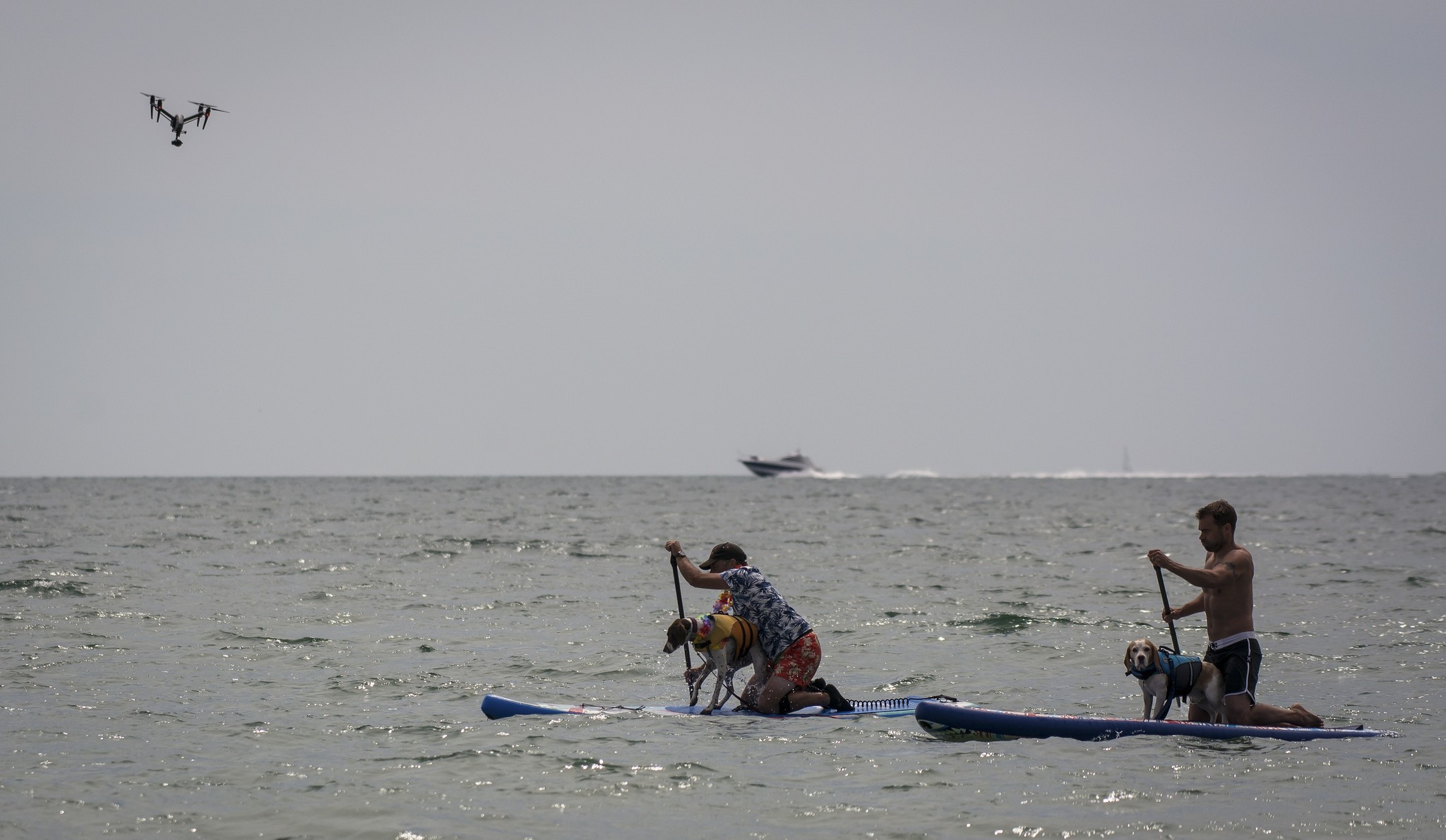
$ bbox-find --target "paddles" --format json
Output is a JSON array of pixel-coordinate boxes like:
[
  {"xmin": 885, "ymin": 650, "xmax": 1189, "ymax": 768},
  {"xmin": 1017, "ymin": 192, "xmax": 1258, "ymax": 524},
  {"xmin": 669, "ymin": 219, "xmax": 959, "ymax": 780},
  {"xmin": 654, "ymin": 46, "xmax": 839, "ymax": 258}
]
[
  {"xmin": 1153, "ymin": 564, "xmax": 1182, "ymax": 656},
  {"xmin": 669, "ymin": 545, "xmax": 695, "ymax": 702}
]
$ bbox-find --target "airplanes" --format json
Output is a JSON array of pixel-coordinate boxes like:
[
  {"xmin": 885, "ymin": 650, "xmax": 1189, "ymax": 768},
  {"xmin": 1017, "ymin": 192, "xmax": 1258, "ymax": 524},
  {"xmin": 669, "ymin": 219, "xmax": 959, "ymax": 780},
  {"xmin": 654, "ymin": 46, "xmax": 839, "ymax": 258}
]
[{"xmin": 139, "ymin": 92, "xmax": 230, "ymax": 147}]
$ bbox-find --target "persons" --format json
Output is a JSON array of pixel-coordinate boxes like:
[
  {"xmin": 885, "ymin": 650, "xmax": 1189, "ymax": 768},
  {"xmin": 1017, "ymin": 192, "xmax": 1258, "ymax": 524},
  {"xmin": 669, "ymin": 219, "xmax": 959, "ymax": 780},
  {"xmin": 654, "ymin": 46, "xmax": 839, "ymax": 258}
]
[
  {"xmin": 1148, "ymin": 499, "xmax": 1325, "ymax": 727},
  {"xmin": 665, "ymin": 540, "xmax": 855, "ymax": 714}
]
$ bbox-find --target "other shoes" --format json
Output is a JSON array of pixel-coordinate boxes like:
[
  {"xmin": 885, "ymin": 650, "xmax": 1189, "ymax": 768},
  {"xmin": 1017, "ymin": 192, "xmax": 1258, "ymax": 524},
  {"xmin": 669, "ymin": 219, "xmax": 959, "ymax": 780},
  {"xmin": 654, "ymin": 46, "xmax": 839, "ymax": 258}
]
[
  {"xmin": 811, "ymin": 677, "xmax": 826, "ymax": 690},
  {"xmin": 821, "ymin": 683, "xmax": 855, "ymax": 712}
]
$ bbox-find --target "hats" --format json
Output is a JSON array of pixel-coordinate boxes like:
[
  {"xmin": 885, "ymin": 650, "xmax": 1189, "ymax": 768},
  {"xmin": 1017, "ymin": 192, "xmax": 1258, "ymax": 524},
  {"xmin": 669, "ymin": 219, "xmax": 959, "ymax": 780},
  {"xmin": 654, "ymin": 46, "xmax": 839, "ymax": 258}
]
[{"xmin": 699, "ymin": 542, "xmax": 748, "ymax": 570}]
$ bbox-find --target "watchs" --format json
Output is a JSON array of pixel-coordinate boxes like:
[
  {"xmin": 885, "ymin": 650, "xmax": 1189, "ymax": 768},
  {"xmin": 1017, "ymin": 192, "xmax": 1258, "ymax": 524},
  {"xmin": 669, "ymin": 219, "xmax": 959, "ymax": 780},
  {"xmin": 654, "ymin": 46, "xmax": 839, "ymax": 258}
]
[{"xmin": 675, "ymin": 551, "xmax": 686, "ymax": 559}]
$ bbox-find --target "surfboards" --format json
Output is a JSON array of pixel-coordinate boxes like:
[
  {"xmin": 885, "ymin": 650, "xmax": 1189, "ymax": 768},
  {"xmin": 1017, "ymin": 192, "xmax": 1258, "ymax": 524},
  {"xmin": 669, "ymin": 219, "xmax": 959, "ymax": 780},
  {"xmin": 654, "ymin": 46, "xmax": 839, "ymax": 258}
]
[
  {"xmin": 480, "ymin": 695, "xmax": 979, "ymax": 721},
  {"xmin": 914, "ymin": 697, "xmax": 1401, "ymax": 742}
]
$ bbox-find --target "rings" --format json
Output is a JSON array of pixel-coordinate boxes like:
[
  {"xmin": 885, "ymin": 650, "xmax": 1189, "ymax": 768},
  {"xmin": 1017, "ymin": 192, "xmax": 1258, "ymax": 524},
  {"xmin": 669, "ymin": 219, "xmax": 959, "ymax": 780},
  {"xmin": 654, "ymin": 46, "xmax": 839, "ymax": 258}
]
[{"xmin": 1152, "ymin": 557, "xmax": 1154, "ymax": 561}]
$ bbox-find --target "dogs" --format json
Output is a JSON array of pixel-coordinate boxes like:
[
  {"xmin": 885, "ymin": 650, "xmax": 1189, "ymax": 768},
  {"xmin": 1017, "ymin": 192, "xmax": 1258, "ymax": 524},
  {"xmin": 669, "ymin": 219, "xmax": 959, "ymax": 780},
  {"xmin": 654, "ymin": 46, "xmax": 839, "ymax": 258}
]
[
  {"xmin": 662, "ymin": 613, "xmax": 770, "ymax": 717},
  {"xmin": 1123, "ymin": 638, "xmax": 1229, "ymax": 725}
]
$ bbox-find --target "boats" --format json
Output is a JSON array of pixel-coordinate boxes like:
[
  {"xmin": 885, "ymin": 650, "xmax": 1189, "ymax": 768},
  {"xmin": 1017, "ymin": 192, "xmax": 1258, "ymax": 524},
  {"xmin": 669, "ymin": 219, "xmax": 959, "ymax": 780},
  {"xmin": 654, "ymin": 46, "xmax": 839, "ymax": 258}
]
[{"xmin": 737, "ymin": 454, "xmax": 826, "ymax": 478}]
